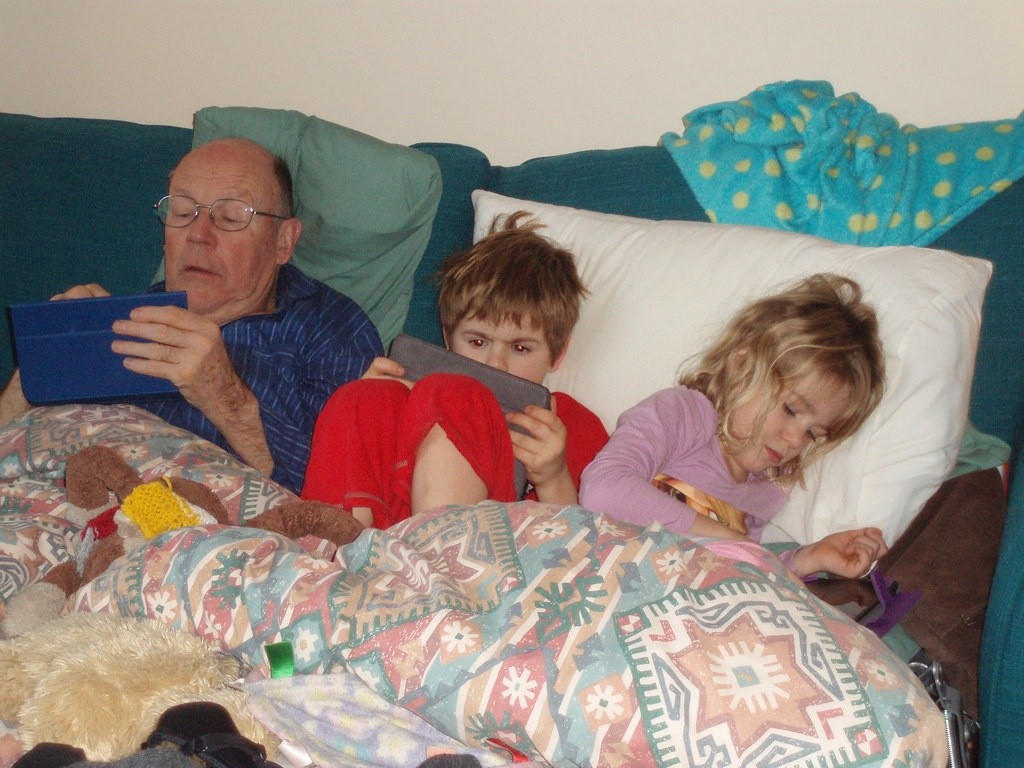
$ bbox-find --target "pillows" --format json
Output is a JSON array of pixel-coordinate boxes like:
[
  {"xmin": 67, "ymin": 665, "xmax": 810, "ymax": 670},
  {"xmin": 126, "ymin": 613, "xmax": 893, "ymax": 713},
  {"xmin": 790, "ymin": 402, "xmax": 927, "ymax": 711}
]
[
  {"xmin": 0, "ymin": 111, "xmax": 493, "ymax": 395},
  {"xmin": 150, "ymin": 105, "xmax": 443, "ymax": 358},
  {"xmin": 487, "ymin": 145, "xmax": 1024, "ymax": 447},
  {"xmin": 469, "ymin": 189, "xmax": 993, "ymax": 579}
]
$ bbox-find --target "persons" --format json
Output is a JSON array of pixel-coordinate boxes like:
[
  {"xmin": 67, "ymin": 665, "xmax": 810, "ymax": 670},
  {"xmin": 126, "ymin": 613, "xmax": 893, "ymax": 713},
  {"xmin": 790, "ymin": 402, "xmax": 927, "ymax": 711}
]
[
  {"xmin": 578, "ymin": 271, "xmax": 888, "ymax": 578},
  {"xmin": 301, "ymin": 209, "xmax": 610, "ymax": 528},
  {"xmin": 0, "ymin": 136, "xmax": 385, "ymax": 505}
]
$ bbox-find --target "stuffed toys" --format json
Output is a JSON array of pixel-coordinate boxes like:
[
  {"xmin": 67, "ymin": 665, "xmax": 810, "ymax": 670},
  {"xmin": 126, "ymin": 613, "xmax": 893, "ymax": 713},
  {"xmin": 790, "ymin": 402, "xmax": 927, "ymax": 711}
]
[
  {"xmin": 3, "ymin": 445, "xmax": 368, "ymax": 638},
  {"xmin": 0, "ymin": 611, "xmax": 294, "ymax": 768}
]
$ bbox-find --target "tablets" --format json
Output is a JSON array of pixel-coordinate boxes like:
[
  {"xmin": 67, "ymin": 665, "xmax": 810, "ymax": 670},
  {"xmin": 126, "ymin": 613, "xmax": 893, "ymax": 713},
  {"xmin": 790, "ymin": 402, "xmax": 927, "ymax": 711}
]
[
  {"xmin": 9, "ymin": 290, "xmax": 188, "ymax": 407},
  {"xmin": 382, "ymin": 334, "xmax": 551, "ymax": 503},
  {"xmin": 801, "ymin": 579, "xmax": 898, "ymax": 624}
]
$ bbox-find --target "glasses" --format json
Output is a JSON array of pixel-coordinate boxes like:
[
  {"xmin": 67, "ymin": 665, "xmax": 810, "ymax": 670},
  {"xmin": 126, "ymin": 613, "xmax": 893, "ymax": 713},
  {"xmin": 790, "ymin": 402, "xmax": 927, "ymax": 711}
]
[{"xmin": 151, "ymin": 194, "xmax": 286, "ymax": 231}]
